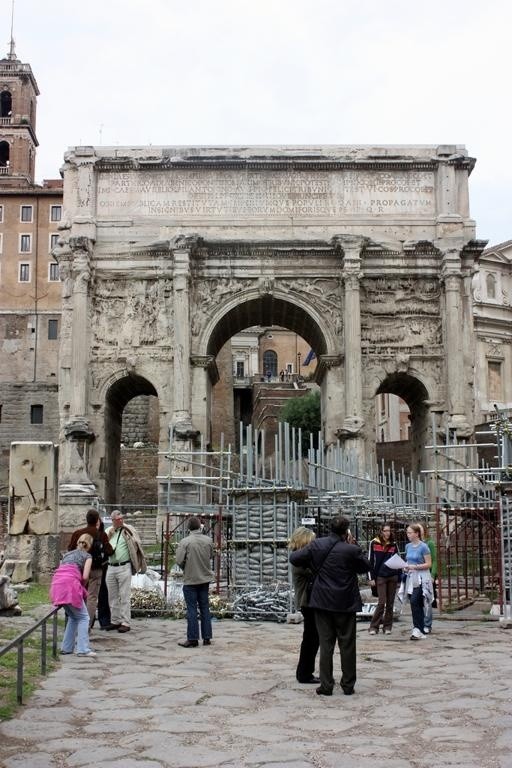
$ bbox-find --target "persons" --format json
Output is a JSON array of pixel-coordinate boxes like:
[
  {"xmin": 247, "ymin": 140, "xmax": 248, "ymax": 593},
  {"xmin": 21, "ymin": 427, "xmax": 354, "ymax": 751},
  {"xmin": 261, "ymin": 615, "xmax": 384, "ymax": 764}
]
[
  {"xmin": 67, "ymin": 509, "xmax": 116, "ymax": 634},
  {"xmin": 98, "ymin": 521, "xmax": 122, "ymax": 632},
  {"xmin": 367, "ymin": 522, "xmax": 402, "ymax": 635},
  {"xmin": 49, "ymin": 533, "xmax": 97, "ymax": 657},
  {"xmin": 106, "ymin": 509, "xmax": 148, "ymax": 633},
  {"xmin": 288, "ymin": 516, "xmax": 371, "ymax": 696},
  {"xmin": 284, "ymin": 526, "xmax": 320, "ymax": 684},
  {"xmin": 284, "ymin": 369, "xmax": 290, "ymax": 383},
  {"xmin": 280, "ymin": 368, "xmax": 285, "ymax": 382},
  {"xmin": 398, "ymin": 524, "xmax": 432, "ymax": 641},
  {"xmin": 266, "ymin": 367, "xmax": 272, "ymax": 383},
  {"xmin": 418, "ymin": 521, "xmax": 438, "ymax": 608},
  {"xmin": 175, "ymin": 516, "xmax": 217, "ymax": 647}
]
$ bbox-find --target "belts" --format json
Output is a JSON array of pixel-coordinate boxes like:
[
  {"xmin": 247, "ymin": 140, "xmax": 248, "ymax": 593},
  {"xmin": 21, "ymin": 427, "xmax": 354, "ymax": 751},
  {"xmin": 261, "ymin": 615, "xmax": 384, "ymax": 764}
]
[{"xmin": 107, "ymin": 559, "xmax": 131, "ymax": 566}]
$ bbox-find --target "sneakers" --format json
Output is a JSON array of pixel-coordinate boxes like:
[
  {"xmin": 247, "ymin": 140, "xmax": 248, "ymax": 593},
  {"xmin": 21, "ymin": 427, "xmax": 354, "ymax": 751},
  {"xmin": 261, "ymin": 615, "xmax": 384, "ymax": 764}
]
[
  {"xmin": 316, "ymin": 684, "xmax": 334, "ymax": 696},
  {"xmin": 410, "ymin": 626, "xmax": 425, "ymax": 640},
  {"xmin": 343, "ymin": 687, "xmax": 355, "ymax": 695},
  {"xmin": 385, "ymin": 628, "xmax": 392, "ymax": 634},
  {"xmin": 76, "ymin": 650, "xmax": 97, "ymax": 657},
  {"xmin": 104, "ymin": 622, "xmax": 131, "ymax": 633},
  {"xmin": 369, "ymin": 630, "xmax": 377, "ymax": 634}
]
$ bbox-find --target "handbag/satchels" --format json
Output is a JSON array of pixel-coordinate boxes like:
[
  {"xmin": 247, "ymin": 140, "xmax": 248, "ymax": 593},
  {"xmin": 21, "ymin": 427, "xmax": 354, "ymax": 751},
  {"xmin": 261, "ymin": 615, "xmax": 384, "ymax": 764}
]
[
  {"xmin": 371, "ymin": 572, "xmax": 379, "ymax": 596},
  {"xmin": 90, "ymin": 539, "xmax": 108, "ymax": 571}
]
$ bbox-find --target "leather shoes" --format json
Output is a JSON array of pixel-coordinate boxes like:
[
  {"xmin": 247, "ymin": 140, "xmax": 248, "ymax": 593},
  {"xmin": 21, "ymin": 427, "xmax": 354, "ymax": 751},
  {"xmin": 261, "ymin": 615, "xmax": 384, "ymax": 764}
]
[
  {"xmin": 297, "ymin": 676, "xmax": 321, "ymax": 684},
  {"xmin": 177, "ymin": 639, "xmax": 199, "ymax": 647},
  {"xmin": 202, "ymin": 638, "xmax": 211, "ymax": 645}
]
